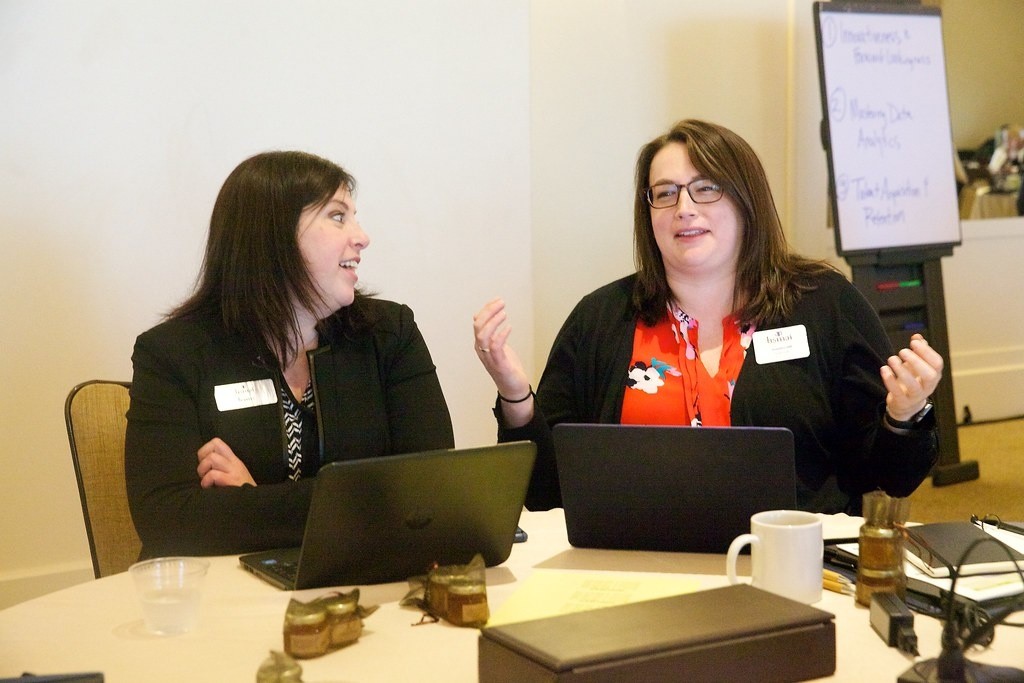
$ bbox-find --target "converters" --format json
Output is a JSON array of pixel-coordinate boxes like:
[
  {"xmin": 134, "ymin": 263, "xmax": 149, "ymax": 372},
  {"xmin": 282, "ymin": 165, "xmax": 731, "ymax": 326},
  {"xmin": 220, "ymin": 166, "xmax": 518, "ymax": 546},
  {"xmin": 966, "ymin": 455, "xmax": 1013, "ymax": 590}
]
[{"xmin": 868, "ymin": 591, "xmax": 914, "ymax": 647}]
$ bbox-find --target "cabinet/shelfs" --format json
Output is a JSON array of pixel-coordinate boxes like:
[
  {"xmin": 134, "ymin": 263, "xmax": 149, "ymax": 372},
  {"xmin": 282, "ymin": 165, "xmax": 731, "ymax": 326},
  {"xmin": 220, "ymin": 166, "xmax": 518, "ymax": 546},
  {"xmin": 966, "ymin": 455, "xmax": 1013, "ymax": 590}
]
[{"xmin": 940, "ymin": 217, "xmax": 1024, "ymax": 425}]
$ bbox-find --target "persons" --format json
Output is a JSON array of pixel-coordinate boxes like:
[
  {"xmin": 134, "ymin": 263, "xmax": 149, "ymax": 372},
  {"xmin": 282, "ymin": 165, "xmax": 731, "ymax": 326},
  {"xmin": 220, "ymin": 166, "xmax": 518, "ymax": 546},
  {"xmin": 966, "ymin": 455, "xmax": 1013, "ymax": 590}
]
[
  {"xmin": 124, "ymin": 150, "xmax": 455, "ymax": 564},
  {"xmin": 472, "ymin": 119, "xmax": 945, "ymax": 522}
]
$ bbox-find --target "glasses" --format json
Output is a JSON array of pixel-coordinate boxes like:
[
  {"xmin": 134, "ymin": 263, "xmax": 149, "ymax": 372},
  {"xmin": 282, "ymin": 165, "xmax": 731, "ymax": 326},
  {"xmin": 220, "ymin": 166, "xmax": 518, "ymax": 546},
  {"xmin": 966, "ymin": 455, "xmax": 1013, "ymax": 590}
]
[{"xmin": 642, "ymin": 177, "xmax": 724, "ymax": 209}]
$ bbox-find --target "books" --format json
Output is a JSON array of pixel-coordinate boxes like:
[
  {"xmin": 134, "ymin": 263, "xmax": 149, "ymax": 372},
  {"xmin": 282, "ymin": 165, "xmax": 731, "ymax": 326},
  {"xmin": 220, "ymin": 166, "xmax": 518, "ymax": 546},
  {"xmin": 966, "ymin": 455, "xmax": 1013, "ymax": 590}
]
[{"xmin": 899, "ymin": 522, "xmax": 1024, "ymax": 578}]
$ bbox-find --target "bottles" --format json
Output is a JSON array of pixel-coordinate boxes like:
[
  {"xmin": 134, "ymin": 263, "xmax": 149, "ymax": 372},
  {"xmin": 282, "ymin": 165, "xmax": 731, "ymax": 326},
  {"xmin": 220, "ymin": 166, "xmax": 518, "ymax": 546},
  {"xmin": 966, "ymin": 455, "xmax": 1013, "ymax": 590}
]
[
  {"xmin": 856, "ymin": 491, "xmax": 909, "ymax": 605},
  {"xmin": 254, "ymin": 566, "xmax": 489, "ymax": 683}
]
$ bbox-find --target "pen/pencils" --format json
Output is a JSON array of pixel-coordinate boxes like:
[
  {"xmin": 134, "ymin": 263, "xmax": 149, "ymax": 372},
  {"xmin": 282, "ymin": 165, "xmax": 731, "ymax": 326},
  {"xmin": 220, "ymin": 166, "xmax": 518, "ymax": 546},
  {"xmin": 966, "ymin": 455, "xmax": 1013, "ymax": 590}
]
[
  {"xmin": 823, "ymin": 578, "xmax": 855, "ymax": 594},
  {"xmin": 823, "ymin": 568, "xmax": 853, "ymax": 583}
]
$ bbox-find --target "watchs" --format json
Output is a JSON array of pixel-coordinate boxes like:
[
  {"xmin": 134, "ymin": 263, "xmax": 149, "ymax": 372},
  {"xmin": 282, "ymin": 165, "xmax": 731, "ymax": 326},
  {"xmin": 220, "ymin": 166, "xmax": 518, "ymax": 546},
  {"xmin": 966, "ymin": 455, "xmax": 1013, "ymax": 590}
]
[{"xmin": 884, "ymin": 400, "xmax": 936, "ymax": 431}]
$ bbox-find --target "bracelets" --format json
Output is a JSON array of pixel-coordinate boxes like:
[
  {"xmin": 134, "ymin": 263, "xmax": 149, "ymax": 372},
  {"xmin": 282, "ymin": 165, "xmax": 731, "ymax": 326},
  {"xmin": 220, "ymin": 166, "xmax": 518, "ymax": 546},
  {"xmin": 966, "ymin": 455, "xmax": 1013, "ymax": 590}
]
[{"xmin": 497, "ymin": 384, "xmax": 532, "ymax": 403}]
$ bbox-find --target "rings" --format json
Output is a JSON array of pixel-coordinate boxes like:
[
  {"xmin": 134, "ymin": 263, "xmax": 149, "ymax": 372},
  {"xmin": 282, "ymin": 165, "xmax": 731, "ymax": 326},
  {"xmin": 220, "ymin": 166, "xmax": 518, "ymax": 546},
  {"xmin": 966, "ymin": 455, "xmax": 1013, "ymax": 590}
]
[{"xmin": 479, "ymin": 346, "xmax": 490, "ymax": 353}]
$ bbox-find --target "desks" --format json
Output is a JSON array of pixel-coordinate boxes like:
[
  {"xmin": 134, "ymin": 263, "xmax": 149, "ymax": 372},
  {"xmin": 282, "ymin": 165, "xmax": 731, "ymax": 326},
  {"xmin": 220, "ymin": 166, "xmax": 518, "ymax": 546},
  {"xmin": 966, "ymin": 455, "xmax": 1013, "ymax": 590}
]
[{"xmin": 0, "ymin": 509, "xmax": 1024, "ymax": 683}]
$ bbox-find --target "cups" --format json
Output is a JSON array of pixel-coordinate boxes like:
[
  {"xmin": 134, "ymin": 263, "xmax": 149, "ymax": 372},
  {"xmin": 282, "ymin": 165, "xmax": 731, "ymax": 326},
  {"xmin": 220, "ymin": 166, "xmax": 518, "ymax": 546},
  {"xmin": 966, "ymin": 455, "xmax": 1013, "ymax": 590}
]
[
  {"xmin": 126, "ymin": 555, "xmax": 193, "ymax": 641},
  {"xmin": 726, "ymin": 507, "xmax": 825, "ymax": 606}
]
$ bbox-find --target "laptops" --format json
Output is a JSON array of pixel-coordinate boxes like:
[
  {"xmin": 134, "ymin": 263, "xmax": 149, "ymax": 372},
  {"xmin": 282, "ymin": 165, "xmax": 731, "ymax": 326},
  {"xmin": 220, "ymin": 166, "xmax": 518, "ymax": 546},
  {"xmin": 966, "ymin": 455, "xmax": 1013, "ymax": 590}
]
[
  {"xmin": 239, "ymin": 439, "xmax": 538, "ymax": 592},
  {"xmin": 552, "ymin": 423, "xmax": 797, "ymax": 555}
]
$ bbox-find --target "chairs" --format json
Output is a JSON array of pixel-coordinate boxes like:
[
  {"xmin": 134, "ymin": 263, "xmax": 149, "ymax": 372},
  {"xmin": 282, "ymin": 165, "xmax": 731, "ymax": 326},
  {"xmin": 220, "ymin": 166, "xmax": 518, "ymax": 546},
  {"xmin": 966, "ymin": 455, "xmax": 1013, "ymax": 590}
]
[{"xmin": 65, "ymin": 379, "xmax": 148, "ymax": 578}]
